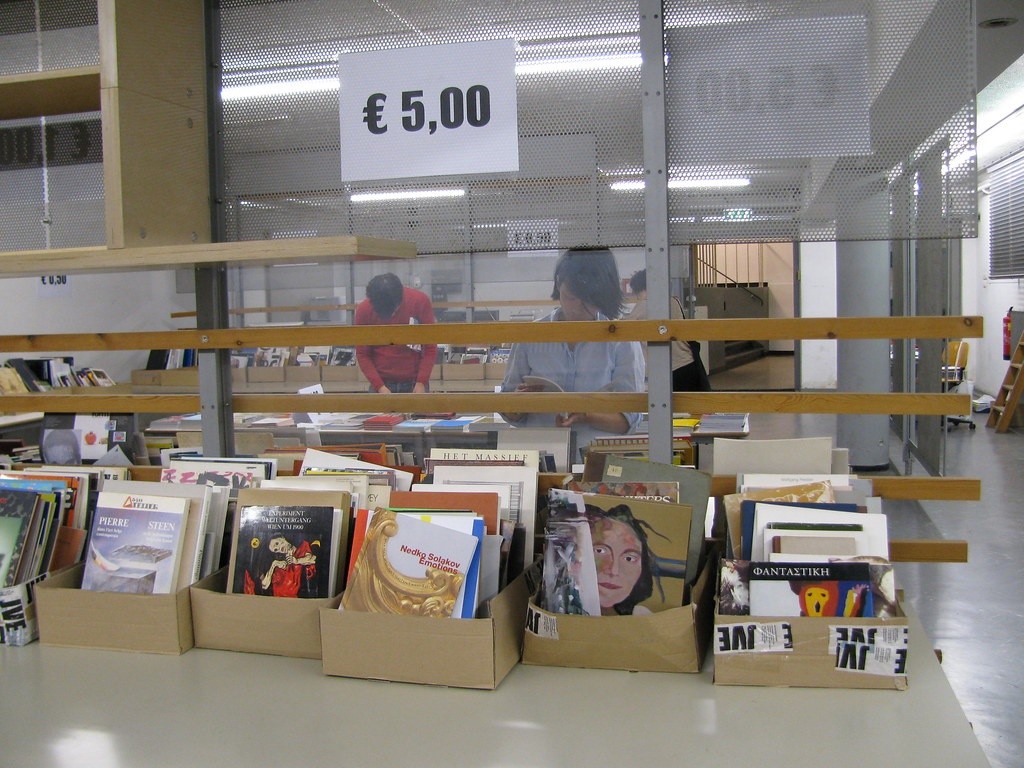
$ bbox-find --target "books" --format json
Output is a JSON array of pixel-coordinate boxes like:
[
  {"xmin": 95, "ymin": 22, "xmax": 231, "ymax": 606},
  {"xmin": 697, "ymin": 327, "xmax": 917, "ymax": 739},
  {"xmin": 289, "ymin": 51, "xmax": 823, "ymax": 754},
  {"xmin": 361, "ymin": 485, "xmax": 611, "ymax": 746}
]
[{"xmin": 0, "ymin": 342, "xmax": 897, "ymax": 647}]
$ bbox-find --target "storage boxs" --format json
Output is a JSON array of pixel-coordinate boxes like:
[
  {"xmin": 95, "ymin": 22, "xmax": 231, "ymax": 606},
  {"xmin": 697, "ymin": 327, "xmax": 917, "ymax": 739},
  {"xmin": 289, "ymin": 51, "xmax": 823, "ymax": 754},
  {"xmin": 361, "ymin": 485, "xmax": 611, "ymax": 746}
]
[
  {"xmin": 520, "ymin": 539, "xmax": 720, "ymax": 668},
  {"xmin": 442, "ymin": 363, "xmax": 487, "ymax": 381},
  {"xmin": 484, "ymin": 361, "xmax": 509, "ymax": 381},
  {"xmin": 190, "ymin": 568, "xmax": 335, "ymax": 662},
  {"xmin": 284, "ymin": 362, "xmax": 323, "ymax": 382},
  {"xmin": 162, "ymin": 365, "xmax": 198, "ymax": 386},
  {"xmin": 247, "ymin": 363, "xmax": 285, "ymax": 383},
  {"xmin": 33, "ymin": 561, "xmax": 221, "ymax": 656},
  {"xmin": 428, "ymin": 360, "xmax": 444, "ymax": 381},
  {"xmin": 711, "ymin": 557, "xmax": 912, "ymax": 691},
  {"xmin": 316, "ymin": 566, "xmax": 534, "ymax": 688},
  {"xmin": 358, "ymin": 365, "xmax": 372, "ymax": 382},
  {"xmin": 1, "ymin": 557, "xmax": 90, "ymax": 647},
  {"xmin": 130, "ymin": 367, "xmax": 164, "ymax": 386},
  {"xmin": 322, "ymin": 363, "xmax": 358, "ymax": 381},
  {"xmin": 232, "ymin": 366, "xmax": 247, "ymax": 384}
]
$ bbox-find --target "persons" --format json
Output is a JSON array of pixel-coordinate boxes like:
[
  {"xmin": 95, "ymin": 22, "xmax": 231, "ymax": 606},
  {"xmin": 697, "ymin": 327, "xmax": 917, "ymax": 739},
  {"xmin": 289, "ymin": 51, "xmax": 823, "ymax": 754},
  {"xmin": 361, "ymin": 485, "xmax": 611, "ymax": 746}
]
[
  {"xmin": 355, "ymin": 271, "xmax": 439, "ymax": 415},
  {"xmin": 620, "ymin": 268, "xmax": 712, "ymax": 391},
  {"xmin": 497, "ymin": 243, "xmax": 646, "ymax": 464}
]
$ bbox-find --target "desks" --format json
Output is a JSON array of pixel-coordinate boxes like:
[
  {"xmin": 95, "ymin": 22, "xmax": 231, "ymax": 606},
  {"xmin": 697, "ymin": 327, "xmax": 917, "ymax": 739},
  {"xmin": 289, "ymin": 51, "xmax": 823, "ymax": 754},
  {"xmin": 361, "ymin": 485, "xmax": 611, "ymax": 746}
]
[
  {"xmin": 889, "ymin": 344, "xmax": 967, "ymax": 393},
  {"xmin": 143, "ymin": 415, "xmax": 496, "ymax": 467},
  {"xmin": 0, "ymin": 595, "xmax": 989, "ymax": 768}
]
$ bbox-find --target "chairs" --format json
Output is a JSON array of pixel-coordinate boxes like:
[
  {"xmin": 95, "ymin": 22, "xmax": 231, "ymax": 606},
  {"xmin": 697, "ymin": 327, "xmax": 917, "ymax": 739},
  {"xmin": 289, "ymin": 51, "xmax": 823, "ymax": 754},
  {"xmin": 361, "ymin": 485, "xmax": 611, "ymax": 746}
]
[{"xmin": 941, "ymin": 340, "xmax": 976, "ymax": 433}]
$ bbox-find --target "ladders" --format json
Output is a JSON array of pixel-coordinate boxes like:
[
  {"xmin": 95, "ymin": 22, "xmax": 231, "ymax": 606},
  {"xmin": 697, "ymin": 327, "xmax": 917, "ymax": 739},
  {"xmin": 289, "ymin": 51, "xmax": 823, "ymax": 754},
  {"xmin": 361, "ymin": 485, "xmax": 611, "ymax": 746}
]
[{"xmin": 985, "ymin": 329, "xmax": 1024, "ymax": 434}]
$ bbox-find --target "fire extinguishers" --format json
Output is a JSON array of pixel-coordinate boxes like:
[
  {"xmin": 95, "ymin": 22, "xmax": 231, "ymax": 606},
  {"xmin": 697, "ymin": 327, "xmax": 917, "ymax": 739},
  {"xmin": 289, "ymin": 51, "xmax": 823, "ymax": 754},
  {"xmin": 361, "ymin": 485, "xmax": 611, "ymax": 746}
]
[{"xmin": 1001, "ymin": 306, "xmax": 1015, "ymax": 360}]
